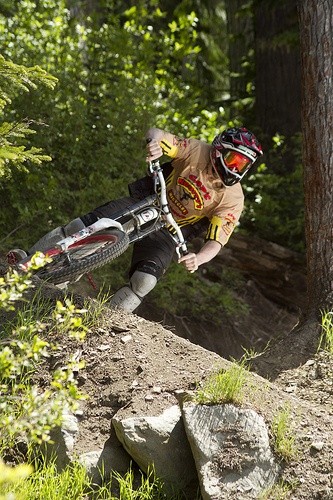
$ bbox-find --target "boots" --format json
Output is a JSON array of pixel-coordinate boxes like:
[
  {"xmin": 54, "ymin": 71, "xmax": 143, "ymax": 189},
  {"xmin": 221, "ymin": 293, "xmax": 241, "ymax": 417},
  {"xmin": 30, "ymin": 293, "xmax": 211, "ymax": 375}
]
[{"xmin": 7, "ymin": 227, "xmax": 62, "ymax": 264}]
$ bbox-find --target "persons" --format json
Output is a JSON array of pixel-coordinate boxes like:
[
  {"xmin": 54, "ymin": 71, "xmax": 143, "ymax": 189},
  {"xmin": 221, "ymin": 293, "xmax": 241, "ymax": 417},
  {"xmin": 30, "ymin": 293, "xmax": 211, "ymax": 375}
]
[{"xmin": 4, "ymin": 126, "xmax": 264, "ymax": 316}]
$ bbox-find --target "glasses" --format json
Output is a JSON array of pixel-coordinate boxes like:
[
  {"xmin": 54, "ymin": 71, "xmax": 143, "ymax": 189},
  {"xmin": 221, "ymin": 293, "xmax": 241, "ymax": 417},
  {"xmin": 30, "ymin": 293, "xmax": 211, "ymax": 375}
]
[{"xmin": 212, "ymin": 137, "xmax": 252, "ymax": 172}]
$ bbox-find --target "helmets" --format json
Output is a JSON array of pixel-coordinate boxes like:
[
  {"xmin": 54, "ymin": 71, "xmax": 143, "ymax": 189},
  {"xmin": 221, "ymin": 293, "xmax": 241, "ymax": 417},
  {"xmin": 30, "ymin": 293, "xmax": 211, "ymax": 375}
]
[{"xmin": 210, "ymin": 128, "xmax": 263, "ymax": 188}]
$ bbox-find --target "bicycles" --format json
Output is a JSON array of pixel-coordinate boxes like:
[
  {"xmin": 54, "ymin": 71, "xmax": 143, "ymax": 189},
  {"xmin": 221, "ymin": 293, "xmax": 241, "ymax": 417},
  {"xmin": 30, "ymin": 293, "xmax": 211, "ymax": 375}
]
[{"xmin": 14, "ymin": 136, "xmax": 194, "ymax": 312}]
